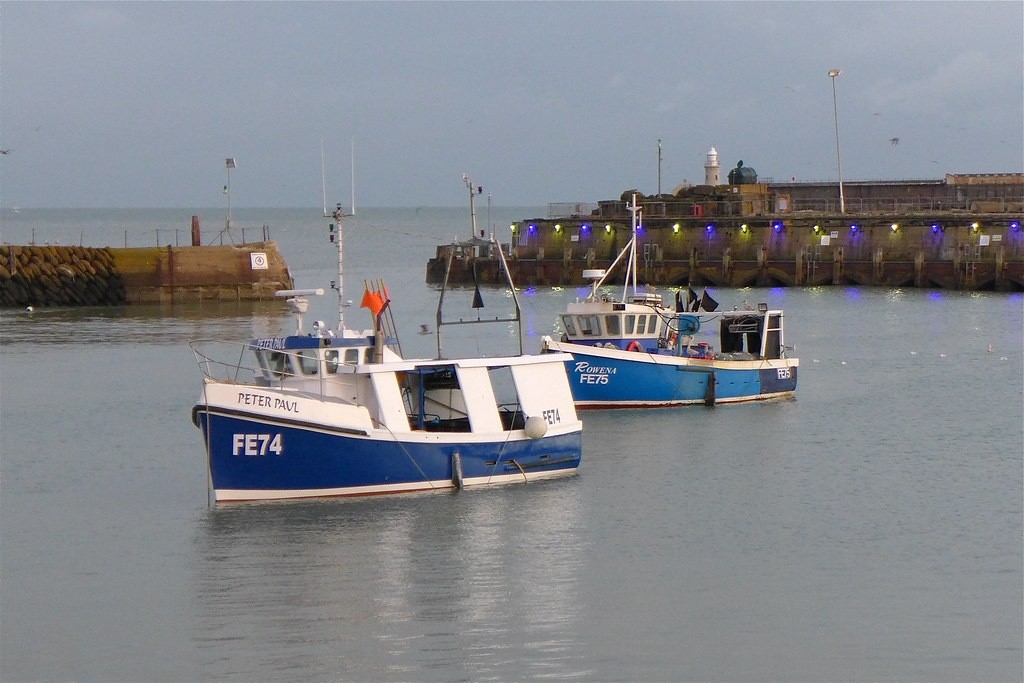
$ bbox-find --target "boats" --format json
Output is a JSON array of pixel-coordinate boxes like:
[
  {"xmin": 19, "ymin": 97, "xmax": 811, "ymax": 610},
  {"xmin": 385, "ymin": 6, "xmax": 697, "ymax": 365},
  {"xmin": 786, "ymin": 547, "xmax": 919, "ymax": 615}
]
[
  {"xmin": 247, "ymin": 141, "xmax": 408, "ymax": 381},
  {"xmin": 538, "ymin": 189, "xmax": 800, "ymax": 409},
  {"xmin": 185, "ymin": 233, "xmax": 586, "ymax": 500}
]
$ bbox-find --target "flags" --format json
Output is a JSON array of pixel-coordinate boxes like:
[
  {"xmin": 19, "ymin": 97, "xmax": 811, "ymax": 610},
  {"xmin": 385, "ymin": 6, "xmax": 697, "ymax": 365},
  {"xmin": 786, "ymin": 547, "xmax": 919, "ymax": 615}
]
[
  {"xmin": 675, "ymin": 287, "xmax": 719, "ymax": 312},
  {"xmin": 360, "ymin": 287, "xmax": 388, "ymax": 314}
]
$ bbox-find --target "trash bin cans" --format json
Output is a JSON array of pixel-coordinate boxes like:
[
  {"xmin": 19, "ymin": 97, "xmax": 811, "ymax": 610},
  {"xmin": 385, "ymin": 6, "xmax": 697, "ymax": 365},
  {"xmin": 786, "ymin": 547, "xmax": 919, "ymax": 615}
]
[{"xmin": 690, "ymin": 205, "xmax": 702, "ymax": 215}]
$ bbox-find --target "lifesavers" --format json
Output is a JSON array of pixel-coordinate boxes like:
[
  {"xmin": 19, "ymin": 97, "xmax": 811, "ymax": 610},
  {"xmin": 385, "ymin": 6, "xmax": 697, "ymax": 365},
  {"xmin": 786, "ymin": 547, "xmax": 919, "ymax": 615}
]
[{"xmin": 627, "ymin": 341, "xmax": 644, "ymax": 353}]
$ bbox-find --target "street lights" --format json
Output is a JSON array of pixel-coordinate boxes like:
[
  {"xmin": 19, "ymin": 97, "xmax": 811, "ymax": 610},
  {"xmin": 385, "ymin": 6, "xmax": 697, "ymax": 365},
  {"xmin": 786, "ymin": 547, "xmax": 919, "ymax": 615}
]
[
  {"xmin": 225, "ymin": 157, "xmax": 237, "ymax": 230},
  {"xmin": 828, "ymin": 69, "xmax": 847, "ymax": 216}
]
